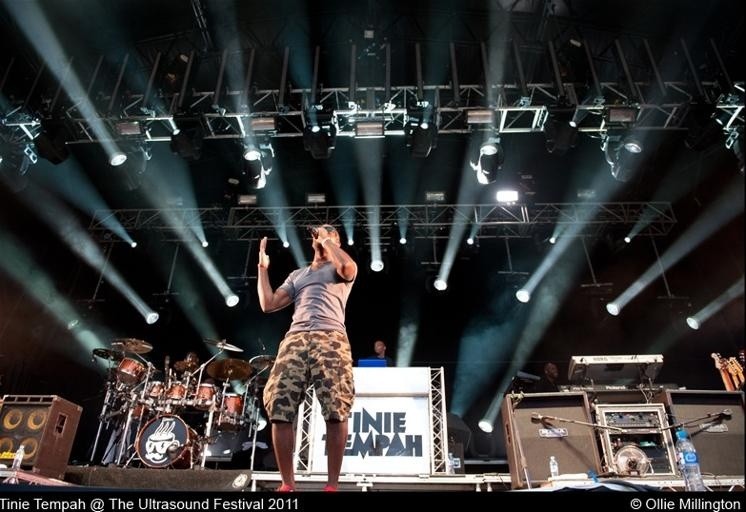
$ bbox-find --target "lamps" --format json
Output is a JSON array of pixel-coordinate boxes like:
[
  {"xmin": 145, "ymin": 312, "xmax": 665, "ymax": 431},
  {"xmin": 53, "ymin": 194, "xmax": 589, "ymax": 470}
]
[{"xmin": 0, "ymin": 33, "xmax": 746, "ymax": 189}]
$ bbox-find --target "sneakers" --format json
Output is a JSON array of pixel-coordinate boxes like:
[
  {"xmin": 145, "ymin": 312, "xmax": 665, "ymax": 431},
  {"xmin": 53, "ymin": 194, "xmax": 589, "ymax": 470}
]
[{"xmin": 276, "ymin": 484, "xmax": 339, "ymax": 492}]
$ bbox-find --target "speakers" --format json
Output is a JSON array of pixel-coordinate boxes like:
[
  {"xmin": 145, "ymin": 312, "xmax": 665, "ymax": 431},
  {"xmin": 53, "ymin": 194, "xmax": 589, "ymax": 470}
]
[
  {"xmin": 500, "ymin": 391, "xmax": 604, "ymax": 490},
  {"xmin": 0, "ymin": 395, "xmax": 84, "ymax": 480},
  {"xmin": 660, "ymin": 389, "xmax": 746, "ymax": 476}
]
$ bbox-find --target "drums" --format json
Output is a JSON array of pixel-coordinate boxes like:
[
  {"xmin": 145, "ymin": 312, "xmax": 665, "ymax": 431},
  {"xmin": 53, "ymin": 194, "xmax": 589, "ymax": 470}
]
[
  {"xmin": 164, "ymin": 381, "xmax": 186, "ymax": 400},
  {"xmin": 131, "ymin": 403, "xmax": 150, "ymax": 422},
  {"xmin": 135, "ymin": 414, "xmax": 202, "ymax": 469},
  {"xmin": 224, "ymin": 393, "xmax": 244, "ymax": 424},
  {"xmin": 192, "ymin": 383, "xmax": 216, "ymax": 411},
  {"xmin": 145, "ymin": 380, "xmax": 166, "ymax": 398},
  {"xmin": 116, "ymin": 357, "xmax": 145, "ymax": 386}
]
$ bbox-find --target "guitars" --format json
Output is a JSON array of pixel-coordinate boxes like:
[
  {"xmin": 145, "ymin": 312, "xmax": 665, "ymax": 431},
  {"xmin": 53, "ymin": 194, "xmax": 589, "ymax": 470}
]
[{"xmin": 711, "ymin": 351, "xmax": 745, "ymax": 391}]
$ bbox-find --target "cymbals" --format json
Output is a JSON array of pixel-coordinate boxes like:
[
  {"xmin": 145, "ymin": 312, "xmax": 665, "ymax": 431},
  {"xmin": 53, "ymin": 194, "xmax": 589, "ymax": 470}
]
[
  {"xmin": 202, "ymin": 337, "xmax": 244, "ymax": 353},
  {"xmin": 206, "ymin": 359, "xmax": 253, "ymax": 380},
  {"xmin": 249, "ymin": 355, "xmax": 276, "ymax": 370},
  {"xmin": 111, "ymin": 338, "xmax": 153, "ymax": 354},
  {"xmin": 92, "ymin": 348, "xmax": 124, "ymax": 361}
]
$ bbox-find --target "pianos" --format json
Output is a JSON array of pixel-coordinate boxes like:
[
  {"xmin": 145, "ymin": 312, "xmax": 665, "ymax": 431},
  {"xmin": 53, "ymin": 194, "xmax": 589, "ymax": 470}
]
[{"xmin": 568, "ymin": 355, "xmax": 664, "ymax": 385}]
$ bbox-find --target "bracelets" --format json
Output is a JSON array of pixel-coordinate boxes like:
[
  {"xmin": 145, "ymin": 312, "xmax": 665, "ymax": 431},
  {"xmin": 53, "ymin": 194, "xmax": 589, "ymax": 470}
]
[
  {"xmin": 321, "ymin": 236, "xmax": 331, "ymax": 247},
  {"xmin": 257, "ymin": 263, "xmax": 269, "ymax": 269}
]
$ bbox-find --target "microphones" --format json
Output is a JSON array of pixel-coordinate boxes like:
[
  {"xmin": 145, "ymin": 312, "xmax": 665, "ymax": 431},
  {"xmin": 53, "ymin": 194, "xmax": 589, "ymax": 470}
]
[
  {"xmin": 168, "ymin": 445, "xmax": 177, "ymax": 453},
  {"xmin": 259, "ymin": 337, "xmax": 266, "ymax": 351},
  {"xmin": 306, "ymin": 224, "xmax": 319, "ymax": 239}
]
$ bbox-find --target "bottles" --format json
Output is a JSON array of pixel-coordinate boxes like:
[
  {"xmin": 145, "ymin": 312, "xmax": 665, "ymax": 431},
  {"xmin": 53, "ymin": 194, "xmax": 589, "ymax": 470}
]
[
  {"xmin": 447, "ymin": 453, "xmax": 456, "ymax": 475},
  {"xmin": 675, "ymin": 431, "xmax": 705, "ymax": 492},
  {"xmin": 11, "ymin": 445, "xmax": 25, "ymax": 471},
  {"xmin": 549, "ymin": 456, "xmax": 559, "ymax": 477}
]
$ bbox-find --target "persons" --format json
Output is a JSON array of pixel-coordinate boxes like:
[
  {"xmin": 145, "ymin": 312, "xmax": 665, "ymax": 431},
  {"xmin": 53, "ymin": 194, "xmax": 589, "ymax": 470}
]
[
  {"xmin": 535, "ymin": 362, "xmax": 559, "ymax": 392},
  {"xmin": 367, "ymin": 340, "xmax": 393, "ymax": 366},
  {"xmin": 256, "ymin": 222, "xmax": 359, "ymax": 491}
]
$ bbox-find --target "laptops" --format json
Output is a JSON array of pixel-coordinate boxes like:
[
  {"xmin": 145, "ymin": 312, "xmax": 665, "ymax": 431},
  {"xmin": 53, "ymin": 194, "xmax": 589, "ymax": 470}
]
[{"xmin": 358, "ymin": 358, "xmax": 387, "ymax": 367}]
